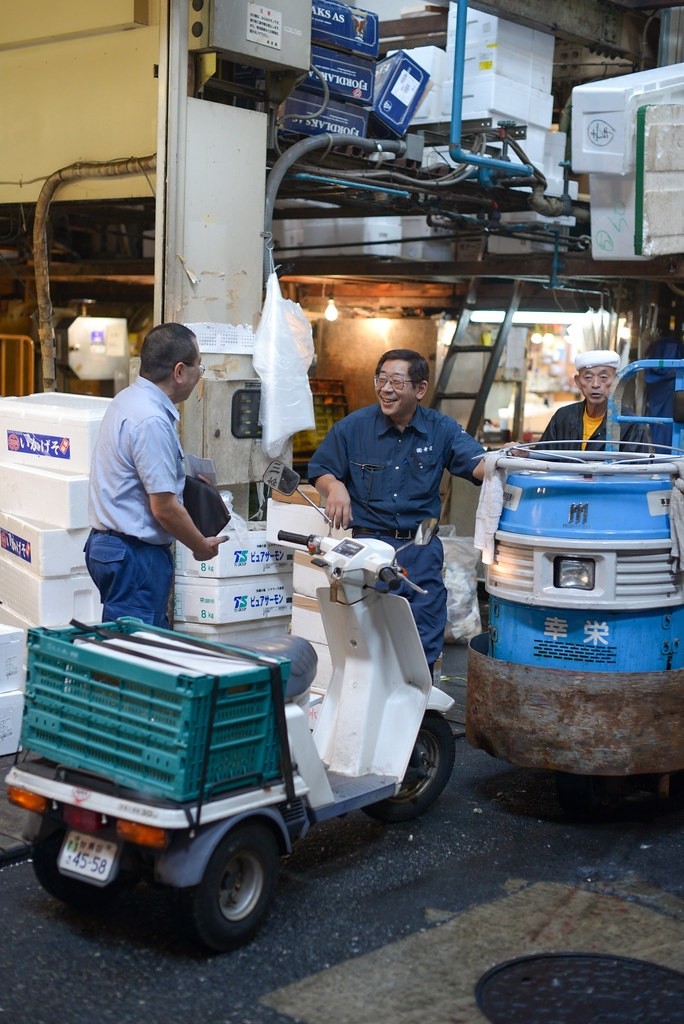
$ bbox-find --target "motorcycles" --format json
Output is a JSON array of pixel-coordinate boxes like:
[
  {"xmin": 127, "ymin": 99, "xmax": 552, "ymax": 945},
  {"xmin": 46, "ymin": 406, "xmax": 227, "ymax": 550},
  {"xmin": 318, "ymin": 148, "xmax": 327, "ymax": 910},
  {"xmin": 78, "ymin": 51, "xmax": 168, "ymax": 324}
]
[{"xmin": 5, "ymin": 456, "xmax": 457, "ymax": 949}]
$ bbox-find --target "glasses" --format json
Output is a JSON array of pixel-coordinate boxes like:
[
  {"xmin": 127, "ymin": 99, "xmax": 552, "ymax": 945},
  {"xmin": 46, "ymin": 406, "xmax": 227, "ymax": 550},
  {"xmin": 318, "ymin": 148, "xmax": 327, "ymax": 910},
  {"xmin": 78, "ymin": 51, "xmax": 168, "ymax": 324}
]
[
  {"xmin": 184, "ymin": 362, "xmax": 205, "ymax": 376},
  {"xmin": 374, "ymin": 375, "xmax": 414, "ymax": 390}
]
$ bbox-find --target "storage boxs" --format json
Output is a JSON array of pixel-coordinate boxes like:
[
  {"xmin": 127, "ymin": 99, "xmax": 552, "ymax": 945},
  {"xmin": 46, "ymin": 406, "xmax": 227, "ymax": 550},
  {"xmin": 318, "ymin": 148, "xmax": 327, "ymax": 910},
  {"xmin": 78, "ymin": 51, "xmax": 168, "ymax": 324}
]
[
  {"xmin": 278, "ymin": 1, "xmax": 684, "ymax": 262},
  {"xmin": 481, "ymin": 391, "xmax": 578, "ymax": 444},
  {"xmin": 0, "ymin": 393, "xmax": 352, "ymax": 802}
]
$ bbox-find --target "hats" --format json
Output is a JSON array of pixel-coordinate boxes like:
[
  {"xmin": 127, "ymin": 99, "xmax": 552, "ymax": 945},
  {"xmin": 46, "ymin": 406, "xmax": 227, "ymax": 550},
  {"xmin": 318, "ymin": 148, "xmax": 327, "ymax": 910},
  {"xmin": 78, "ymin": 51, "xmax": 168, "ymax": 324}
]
[{"xmin": 574, "ymin": 350, "xmax": 620, "ymax": 372}]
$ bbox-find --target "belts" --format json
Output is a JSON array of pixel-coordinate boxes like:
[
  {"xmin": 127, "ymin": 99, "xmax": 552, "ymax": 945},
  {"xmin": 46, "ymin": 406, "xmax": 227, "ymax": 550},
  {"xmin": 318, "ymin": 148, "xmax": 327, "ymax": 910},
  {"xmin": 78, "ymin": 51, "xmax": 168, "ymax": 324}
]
[
  {"xmin": 352, "ymin": 527, "xmax": 417, "ymax": 540},
  {"xmin": 94, "ymin": 529, "xmax": 173, "ymax": 550}
]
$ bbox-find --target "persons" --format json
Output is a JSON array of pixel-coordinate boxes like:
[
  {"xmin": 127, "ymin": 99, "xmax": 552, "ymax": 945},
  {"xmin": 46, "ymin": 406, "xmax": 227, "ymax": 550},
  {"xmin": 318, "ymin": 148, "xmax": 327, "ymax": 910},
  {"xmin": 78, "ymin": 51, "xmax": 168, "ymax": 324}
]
[
  {"xmin": 82, "ymin": 323, "xmax": 231, "ymax": 630},
  {"xmin": 306, "ymin": 349, "xmax": 531, "ymax": 686},
  {"xmin": 534, "ymin": 350, "xmax": 650, "ymax": 465}
]
